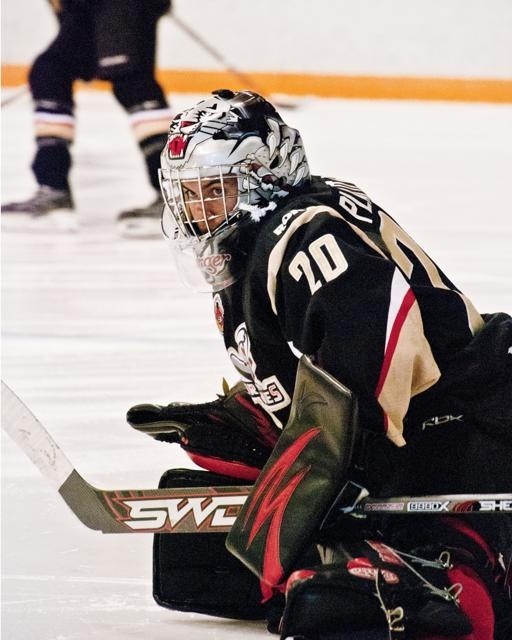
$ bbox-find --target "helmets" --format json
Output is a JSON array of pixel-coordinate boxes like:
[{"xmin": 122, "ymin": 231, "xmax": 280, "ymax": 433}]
[{"xmin": 157, "ymin": 89, "xmax": 311, "ymax": 293}]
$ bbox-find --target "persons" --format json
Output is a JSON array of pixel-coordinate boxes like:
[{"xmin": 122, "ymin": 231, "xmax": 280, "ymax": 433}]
[
  {"xmin": 0, "ymin": 2, "xmax": 180, "ymax": 222},
  {"xmin": 125, "ymin": 87, "xmax": 511, "ymax": 638}
]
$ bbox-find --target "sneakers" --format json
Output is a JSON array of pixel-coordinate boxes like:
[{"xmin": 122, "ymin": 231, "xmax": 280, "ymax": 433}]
[
  {"xmin": 2, "ymin": 185, "xmax": 72, "ymax": 212},
  {"xmin": 121, "ymin": 197, "xmax": 164, "ymax": 217}
]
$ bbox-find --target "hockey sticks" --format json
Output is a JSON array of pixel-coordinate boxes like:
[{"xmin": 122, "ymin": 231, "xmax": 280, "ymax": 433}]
[
  {"xmin": 1, "ymin": 382, "xmax": 511, "ymax": 534},
  {"xmin": 169, "ymin": 8, "xmax": 299, "ymax": 111}
]
